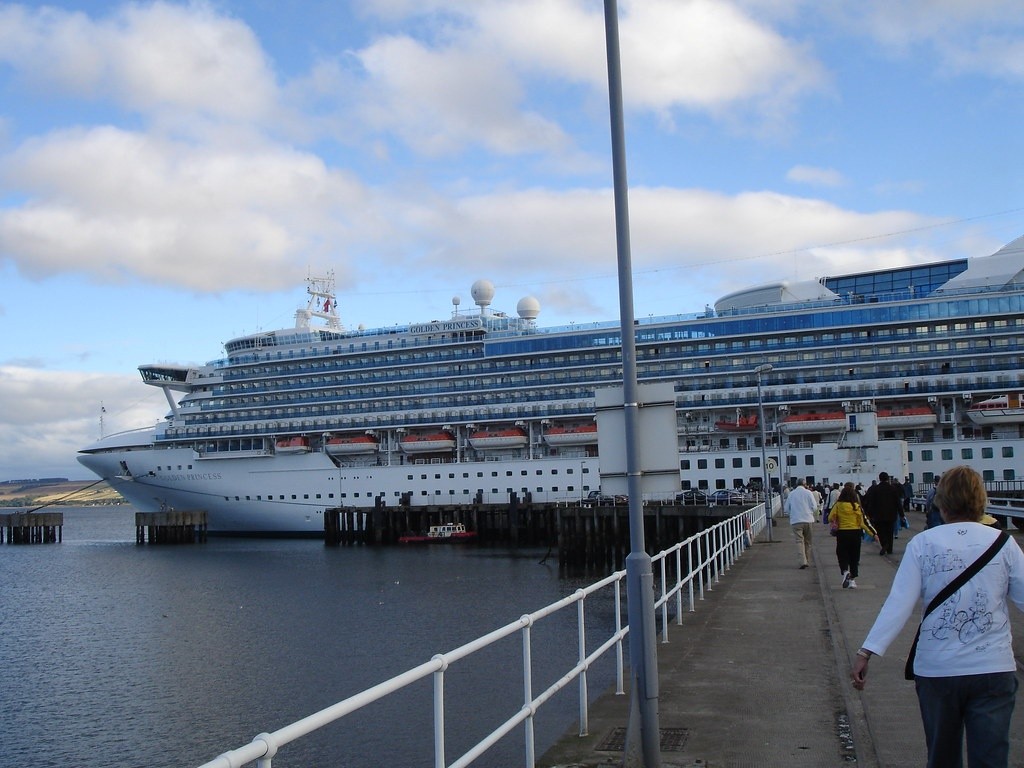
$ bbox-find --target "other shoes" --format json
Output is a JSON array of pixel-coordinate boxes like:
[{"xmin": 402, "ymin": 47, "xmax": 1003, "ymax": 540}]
[{"xmin": 799, "ymin": 565, "xmax": 809, "ymax": 569}]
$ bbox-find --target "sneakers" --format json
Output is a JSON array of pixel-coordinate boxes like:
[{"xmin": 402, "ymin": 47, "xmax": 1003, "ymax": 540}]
[
  {"xmin": 880, "ymin": 545, "xmax": 892, "ymax": 555},
  {"xmin": 848, "ymin": 579, "xmax": 857, "ymax": 589},
  {"xmin": 842, "ymin": 571, "xmax": 850, "ymax": 588}
]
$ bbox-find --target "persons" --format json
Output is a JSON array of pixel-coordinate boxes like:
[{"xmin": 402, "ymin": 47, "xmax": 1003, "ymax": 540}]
[
  {"xmin": 868, "ymin": 472, "xmax": 907, "ymax": 557},
  {"xmin": 733, "ymin": 474, "xmax": 944, "ymax": 538},
  {"xmin": 850, "ymin": 464, "xmax": 1024, "ymax": 768},
  {"xmin": 783, "ymin": 478, "xmax": 818, "ymax": 570},
  {"xmin": 826, "ymin": 485, "xmax": 878, "ymax": 589},
  {"xmin": 977, "ymin": 514, "xmax": 1002, "ymax": 530}
]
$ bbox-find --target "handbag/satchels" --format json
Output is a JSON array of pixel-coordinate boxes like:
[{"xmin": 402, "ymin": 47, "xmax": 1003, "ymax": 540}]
[
  {"xmin": 830, "ymin": 506, "xmax": 839, "ymax": 535},
  {"xmin": 905, "ymin": 623, "xmax": 921, "ymax": 680}
]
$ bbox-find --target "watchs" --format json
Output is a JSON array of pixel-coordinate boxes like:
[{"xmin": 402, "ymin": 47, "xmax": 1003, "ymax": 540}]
[{"xmin": 857, "ymin": 649, "xmax": 871, "ymax": 660}]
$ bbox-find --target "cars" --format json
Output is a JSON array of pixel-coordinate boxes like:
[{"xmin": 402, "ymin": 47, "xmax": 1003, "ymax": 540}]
[
  {"xmin": 707, "ymin": 490, "xmax": 746, "ymax": 505},
  {"xmin": 577, "ymin": 490, "xmax": 628, "ymax": 507},
  {"xmin": 676, "ymin": 491, "xmax": 709, "ymax": 506}
]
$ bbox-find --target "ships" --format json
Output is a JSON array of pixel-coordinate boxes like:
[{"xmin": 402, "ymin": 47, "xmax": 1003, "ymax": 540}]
[{"xmin": 76, "ymin": 236, "xmax": 1024, "ymax": 532}]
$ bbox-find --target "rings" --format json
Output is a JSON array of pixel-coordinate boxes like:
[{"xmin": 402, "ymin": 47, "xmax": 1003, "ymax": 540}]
[{"xmin": 852, "ymin": 680, "xmax": 855, "ymax": 684}]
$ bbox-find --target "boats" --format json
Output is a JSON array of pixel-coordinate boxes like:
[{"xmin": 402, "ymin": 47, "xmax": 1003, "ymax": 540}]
[{"xmin": 398, "ymin": 523, "xmax": 477, "ymax": 544}]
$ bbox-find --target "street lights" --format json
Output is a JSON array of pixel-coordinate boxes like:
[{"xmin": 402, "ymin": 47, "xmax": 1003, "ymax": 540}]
[
  {"xmin": 755, "ymin": 363, "xmax": 775, "ymax": 545},
  {"xmin": 777, "ymin": 423, "xmax": 787, "ymax": 517},
  {"xmin": 784, "ymin": 442, "xmax": 792, "ymax": 492}
]
